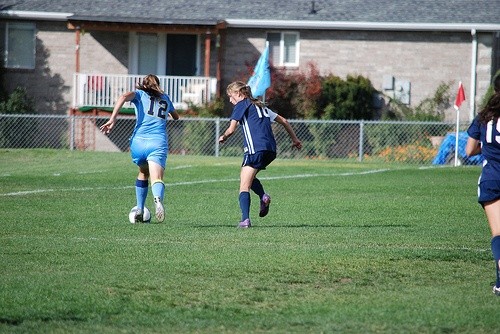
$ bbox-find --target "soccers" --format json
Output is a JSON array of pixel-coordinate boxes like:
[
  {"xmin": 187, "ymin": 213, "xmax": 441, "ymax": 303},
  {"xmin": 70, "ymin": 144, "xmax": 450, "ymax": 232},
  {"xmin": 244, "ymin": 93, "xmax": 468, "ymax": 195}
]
[{"xmin": 129, "ymin": 205, "xmax": 151, "ymax": 224}]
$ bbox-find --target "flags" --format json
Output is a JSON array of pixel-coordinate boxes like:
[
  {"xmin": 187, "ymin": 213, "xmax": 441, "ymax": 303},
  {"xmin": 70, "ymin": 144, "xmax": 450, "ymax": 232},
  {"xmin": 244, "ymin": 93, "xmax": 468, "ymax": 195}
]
[
  {"xmin": 244, "ymin": 42, "xmax": 271, "ymax": 100},
  {"xmin": 454, "ymin": 85, "xmax": 466, "ymax": 109}
]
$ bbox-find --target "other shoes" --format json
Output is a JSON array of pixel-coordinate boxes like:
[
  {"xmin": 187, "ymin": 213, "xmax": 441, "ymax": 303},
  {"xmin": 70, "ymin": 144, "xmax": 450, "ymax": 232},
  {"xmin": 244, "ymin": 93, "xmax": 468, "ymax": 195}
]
[{"xmin": 492, "ymin": 285, "xmax": 500, "ymax": 295}]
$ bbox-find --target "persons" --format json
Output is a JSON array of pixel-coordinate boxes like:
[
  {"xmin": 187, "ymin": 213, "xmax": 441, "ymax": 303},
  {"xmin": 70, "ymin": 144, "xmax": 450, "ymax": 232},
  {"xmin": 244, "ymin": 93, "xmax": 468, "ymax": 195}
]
[
  {"xmin": 99, "ymin": 74, "xmax": 180, "ymax": 224},
  {"xmin": 464, "ymin": 71, "xmax": 500, "ymax": 296},
  {"xmin": 218, "ymin": 81, "xmax": 302, "ymax": 229}
]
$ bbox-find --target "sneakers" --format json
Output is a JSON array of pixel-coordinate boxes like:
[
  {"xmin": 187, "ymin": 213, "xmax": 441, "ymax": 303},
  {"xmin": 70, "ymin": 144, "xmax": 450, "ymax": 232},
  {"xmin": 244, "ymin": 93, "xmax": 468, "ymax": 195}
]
[
  {"xmin": 238, "ymin": 218, "xmax": 251, "ymax": 228},
  {"xmin": 259, "ymin": 193, "xmax": 271, "ymax": 217},
  {"xmin": 153, "ymin": 195, "xmax": 165, "ymax": 223},
  {"xmin": 134, "ymin": 209, "xmax": 144, "ymax": 223}
]
